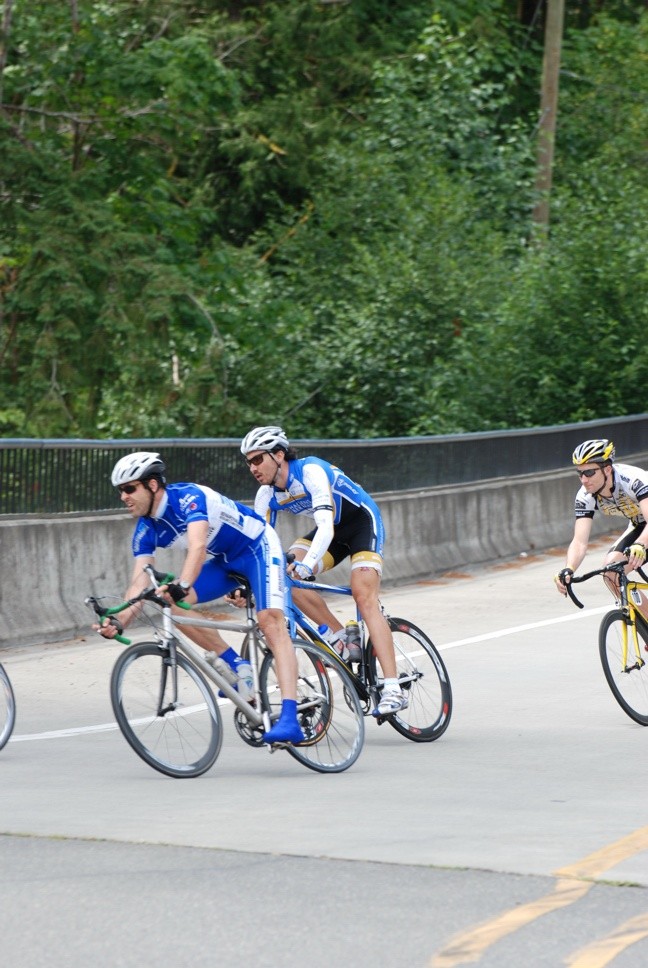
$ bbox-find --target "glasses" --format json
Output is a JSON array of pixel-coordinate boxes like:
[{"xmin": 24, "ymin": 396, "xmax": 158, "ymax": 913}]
[
  {"xmin": 245, "ymin": 450, "xmax": 278, "ymax": 466},
  {"xmin": 117, "ymin": 479, "xmax": 148, "ymax": 494},
  {"xmin": 576, "ymin": 464, "xmax": 610, "ymax": 477}
]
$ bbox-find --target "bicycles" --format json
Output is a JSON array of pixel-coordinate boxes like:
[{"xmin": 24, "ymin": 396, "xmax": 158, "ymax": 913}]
[
  {"xmin": 227, "ymin": 552, "xmax": 453, "ymax": 743},
  {"xmin": 0, "ymin": 663, "xmax": 16, "ymax": 752},
  {"xmin": 82, "ymin": 564, "xmax": 365, "ymax": 778},
  {"xmin": 561, "ymin": 547, "xmax": 648, "ymax": 728}
]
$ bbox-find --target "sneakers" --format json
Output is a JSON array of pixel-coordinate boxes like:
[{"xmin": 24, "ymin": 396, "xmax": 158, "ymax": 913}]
[
  {"xmin": 218, "ymin": 660, "xmax": 256, "ymax": 697},
  {"xmin": 262, "ymin": 716, "xmax": 305, "ymax": 743},
  {"xmin": 321, "ymin": 630, "xmax": 353, "ymax": 665},
  {"xmin": 372, "ymin": 688, "xmax": 408, "ymax": 717}
]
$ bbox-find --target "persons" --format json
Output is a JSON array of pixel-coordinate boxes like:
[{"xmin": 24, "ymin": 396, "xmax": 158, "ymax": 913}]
[
  {"xmin": 554, "ymin": 438, "xmax": 648, "ymax": 651},
  {"xmin": 92, "ymin": 453, "xmax": 306, "ymax": 745},
  {"xmin": 224, "ymin": 426, "xmax": 409, "ymax": 717}
]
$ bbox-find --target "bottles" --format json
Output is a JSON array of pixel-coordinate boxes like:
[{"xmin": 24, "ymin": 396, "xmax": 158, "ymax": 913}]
[
  {"xmin": 204, "ymin": 650, "xmax": 241, "ymax": 686},
  {"xmin": 317, "ymin": 624, "xmax": 350, "ymax": 661},
  {"xmin": 236, "ymin": 659, "xmax": 255, "ymax": 703},
  {"xmin": 343, "ymin": 620, "xmax": 362, "ymax": 662}
]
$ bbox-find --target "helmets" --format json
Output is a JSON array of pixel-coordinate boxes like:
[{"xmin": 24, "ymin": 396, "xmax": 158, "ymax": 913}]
[
  {"xmin": 572, "ymin": 439, "xmax": 616, "ymax": 466},
  {"xmin": 240, "ymin": 426, "xmax": 290, "ymax": 455},
  {"xmin": 110, "ymin": 452, "xmax": 166, "ymax": 487}
]
